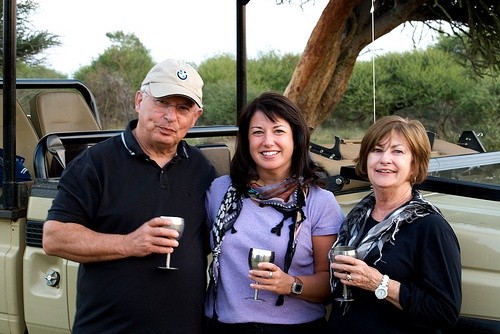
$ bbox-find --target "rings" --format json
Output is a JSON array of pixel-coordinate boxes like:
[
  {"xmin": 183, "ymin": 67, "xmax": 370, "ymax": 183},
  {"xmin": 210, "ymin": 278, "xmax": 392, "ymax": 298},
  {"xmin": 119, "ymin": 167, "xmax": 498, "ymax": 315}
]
[
  {"xmin": 347, "ymin": 275, "xmax": 351, "ymax": 280},
  {"xmin": 269, "ymin": 272, "xmax": 272, "ymax": 279}
]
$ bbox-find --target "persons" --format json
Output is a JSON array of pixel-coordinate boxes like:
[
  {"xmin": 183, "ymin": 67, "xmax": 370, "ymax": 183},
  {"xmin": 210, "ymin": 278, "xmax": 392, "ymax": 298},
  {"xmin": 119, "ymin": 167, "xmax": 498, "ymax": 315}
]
[
  {"xmin": 43, "ymin": 59, "xmax": 218, "ymax": 334},
  {"xmin": 205, "ymin": 92, "xmax": 345, "ymax": 334},
  {"xmin": 326, "ymin": 115, "xmax": 462, "ymax": 334}
]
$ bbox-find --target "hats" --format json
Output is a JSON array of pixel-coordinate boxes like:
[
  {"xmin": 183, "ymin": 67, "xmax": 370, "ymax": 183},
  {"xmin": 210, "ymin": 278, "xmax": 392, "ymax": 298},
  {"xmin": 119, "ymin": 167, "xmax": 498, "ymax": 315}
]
[{"xmin": 142, "ymin": 60, "xmax": 204, "ymax": 108}]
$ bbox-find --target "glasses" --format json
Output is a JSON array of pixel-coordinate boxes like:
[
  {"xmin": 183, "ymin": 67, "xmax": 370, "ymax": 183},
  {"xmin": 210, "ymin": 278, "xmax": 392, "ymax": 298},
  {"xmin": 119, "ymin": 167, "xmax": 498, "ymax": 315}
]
[{"xmin": 142, "ymin": 90, "xmax": 197, "ymax": 118}]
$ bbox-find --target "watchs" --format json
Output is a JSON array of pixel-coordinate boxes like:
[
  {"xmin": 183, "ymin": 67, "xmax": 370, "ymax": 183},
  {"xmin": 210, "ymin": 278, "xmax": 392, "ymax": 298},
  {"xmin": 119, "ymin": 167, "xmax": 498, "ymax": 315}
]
[
  {"xmin": 289, "ymin": 276, "xmax": 303, "ymax": 297},
  {"xmin": 375, "ymin": 275, "xmax": 389, "ymax": 299}
]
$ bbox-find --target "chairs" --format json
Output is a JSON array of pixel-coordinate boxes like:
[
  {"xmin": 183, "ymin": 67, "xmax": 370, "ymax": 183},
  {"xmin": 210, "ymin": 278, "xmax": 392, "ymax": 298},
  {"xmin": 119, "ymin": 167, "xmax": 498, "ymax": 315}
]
[
  {"xmin": 195, "ymin": 143, "xmax": 231, "ymax": 180},
  {"xmin": 30, "ymin": 92, "xmax": 100, "ymax": 169},
  {"xmin": 0, "ymin": 92, "xmax": 47, "ymax": 177}
]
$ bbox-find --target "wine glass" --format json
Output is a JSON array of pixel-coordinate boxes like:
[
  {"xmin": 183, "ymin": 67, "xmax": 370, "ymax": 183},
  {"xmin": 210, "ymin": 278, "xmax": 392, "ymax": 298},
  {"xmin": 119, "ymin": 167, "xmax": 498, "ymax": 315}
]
[
  {"xmin": 333, "ymin": 246, "xmax": 357, "ymax": 302},
  {"xmin": 243, "ymin": 248, "xmax": 275, "ymax": 303},
  {"xmin": 158, "ymin": 215, "xmax": 184, "ymax": 270}
]
